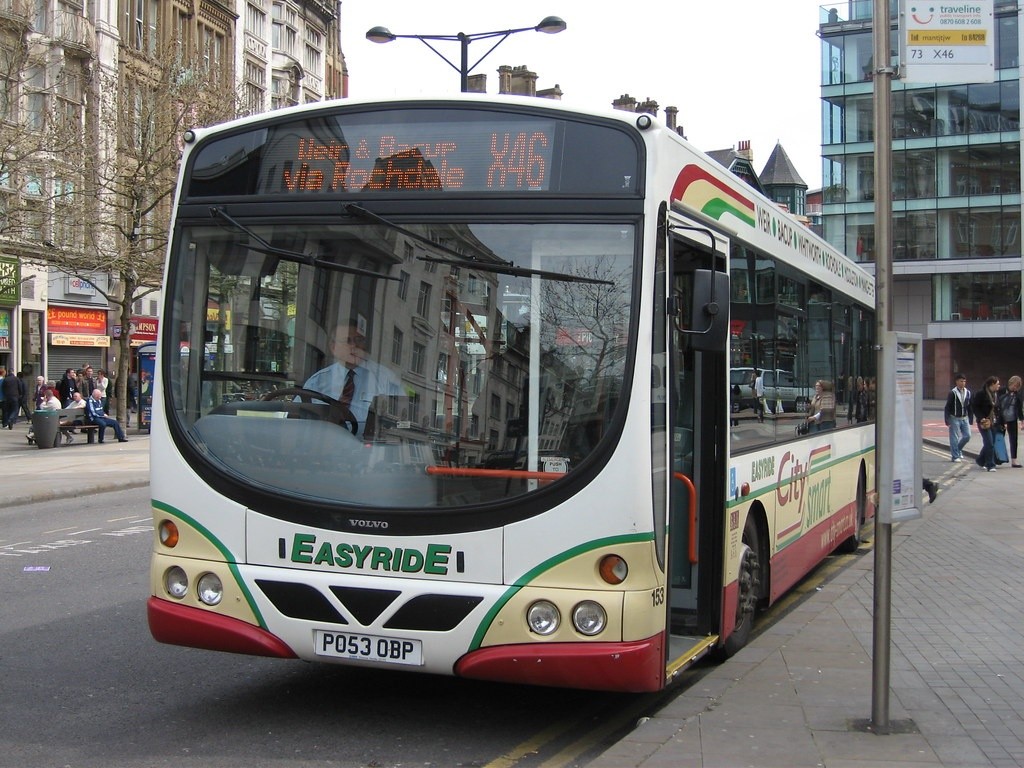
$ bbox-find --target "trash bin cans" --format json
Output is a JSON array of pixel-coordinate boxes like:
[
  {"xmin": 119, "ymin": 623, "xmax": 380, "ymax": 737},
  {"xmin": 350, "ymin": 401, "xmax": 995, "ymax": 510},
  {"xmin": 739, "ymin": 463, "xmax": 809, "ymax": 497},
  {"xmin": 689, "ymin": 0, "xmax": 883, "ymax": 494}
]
[{"xmin": 31, "ymin": 410, "xmax": 62, "ymax": 449}]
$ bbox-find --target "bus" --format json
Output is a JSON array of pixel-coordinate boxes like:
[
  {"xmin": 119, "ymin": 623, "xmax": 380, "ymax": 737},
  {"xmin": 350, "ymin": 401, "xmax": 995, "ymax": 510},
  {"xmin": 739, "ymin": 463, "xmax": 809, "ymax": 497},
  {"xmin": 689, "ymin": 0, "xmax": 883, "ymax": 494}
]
[{"xmin": 141, "ymin": 92, "xmax": 875, "ymax": 697}]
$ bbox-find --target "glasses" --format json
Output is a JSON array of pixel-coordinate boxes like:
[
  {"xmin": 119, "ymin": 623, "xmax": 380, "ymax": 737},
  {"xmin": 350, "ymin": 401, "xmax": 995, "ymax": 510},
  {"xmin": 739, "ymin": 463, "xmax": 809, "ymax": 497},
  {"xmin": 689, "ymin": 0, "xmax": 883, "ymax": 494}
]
[{"xmin": 333, "ymin": 335, "xmax": 364, "ymax": 346}]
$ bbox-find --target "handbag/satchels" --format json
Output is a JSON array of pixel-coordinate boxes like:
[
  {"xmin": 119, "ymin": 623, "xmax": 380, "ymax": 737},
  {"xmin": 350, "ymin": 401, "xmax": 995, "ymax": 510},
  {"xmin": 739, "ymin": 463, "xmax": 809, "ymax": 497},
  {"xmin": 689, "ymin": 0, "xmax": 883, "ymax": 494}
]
[{"xmin": 980, "ymin": 419, "xmax": 990, "ymax": 429}]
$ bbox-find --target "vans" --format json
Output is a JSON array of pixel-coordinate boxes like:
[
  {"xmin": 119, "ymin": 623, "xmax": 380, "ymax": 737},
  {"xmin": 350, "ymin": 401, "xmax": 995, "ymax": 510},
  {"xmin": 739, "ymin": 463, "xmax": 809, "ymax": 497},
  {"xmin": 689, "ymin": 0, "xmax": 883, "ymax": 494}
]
[{"xmin": 730, "ymin": 365, "xmax": 816, "ymax": 413}]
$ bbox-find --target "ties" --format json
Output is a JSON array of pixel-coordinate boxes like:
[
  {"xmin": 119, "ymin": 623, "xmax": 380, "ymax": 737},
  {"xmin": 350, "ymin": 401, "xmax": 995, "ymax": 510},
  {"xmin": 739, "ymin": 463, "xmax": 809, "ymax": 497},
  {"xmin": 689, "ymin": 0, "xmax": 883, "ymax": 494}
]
[{"xmin": 338, "ymin": 370, "xmax": 356, "ymax": 410}]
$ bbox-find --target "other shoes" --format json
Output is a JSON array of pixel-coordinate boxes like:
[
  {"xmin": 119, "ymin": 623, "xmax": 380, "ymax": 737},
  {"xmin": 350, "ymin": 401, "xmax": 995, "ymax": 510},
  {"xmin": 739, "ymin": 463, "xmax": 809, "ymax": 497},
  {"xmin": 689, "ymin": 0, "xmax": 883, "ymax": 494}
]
[
  {"xmin": 7, "ymin": 420, "xmax": 12, "ymax": 429},
  {"xmin": 26, "ymin": 432, "xmax": 35, "ymax": 440},
  {"xmin": 98, "ymin": 439, "xmax": 104, "ymax": 443},
  {"xmin": 980, "ymin": 465, "xmax": 987, "ymax": 470},
  {"xmin": 119, "ymin": 439, "xmax": 128, "ymax": 442},
  {"xmin": 66, "ymin": 437, "xmax": 73, "ymax": 446},
  {"xmin": 959, "ymin": 456, "xmax": 964, "ymax": 459},
  {"xmin": 988, "ymin": 468, "xmax": 997, "ymax": 472},
  {"xmin": 1012, "ymin": 464, "xmax": 1023, "ymax": 467},
  {"xmin": 951, "ymin": 458, "xmax": 961, "ymax": 463}
]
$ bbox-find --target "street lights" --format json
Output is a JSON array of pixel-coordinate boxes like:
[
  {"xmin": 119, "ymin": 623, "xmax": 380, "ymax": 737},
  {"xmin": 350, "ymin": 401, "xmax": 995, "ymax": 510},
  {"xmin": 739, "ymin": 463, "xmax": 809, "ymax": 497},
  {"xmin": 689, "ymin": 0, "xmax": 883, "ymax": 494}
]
[{"xmin": 364, "ymin": 15, "xmax": 567, "ymax": 93}]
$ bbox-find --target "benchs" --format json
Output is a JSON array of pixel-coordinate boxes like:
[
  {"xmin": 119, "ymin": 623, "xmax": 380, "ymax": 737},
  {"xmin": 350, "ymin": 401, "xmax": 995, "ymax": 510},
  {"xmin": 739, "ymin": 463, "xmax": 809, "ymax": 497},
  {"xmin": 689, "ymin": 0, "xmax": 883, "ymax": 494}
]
[{"xmin": 29, "ymin": 408, "xmax": 99, "ymax": 444}]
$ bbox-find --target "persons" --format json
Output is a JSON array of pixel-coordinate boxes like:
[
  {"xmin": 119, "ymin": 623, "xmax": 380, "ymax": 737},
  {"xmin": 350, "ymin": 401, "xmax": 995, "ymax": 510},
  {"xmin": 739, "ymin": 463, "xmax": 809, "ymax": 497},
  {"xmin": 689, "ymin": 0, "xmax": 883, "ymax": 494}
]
[
  {"xmin": 750, "ymin": 365, "xmax": 764, "ymax": 418},
  {"xmin": 972, "ymin": 375, "xmax": 1008, "ymax": 472},
  {"xmin": 290, "ymin": 319, "xmax": 412, "ymax": 442},
  {"xmin": 922, "ymin": 477, "xmax": 940, "ymax": 504},
  {"xmin": 1011, "ymin": 283, "xmax": 1021, "ymax": 320},
  {"xmin": 998, "ymin": 375, "xmax": 1024, "ymax": 468},
  {"xmin": 944, "ymin": 373, "xmax": 974, "ymax": 463},
  {"xmin": 86, "ymin": 389, "xmax": 130, "ymax": 443},
  {"xmin": 114, "ymin": 365, "xmax": 136, "ymax": 427},
  {"xmin": 0, "ymin": 361, "xmax": 113, "ymax": 447},
  {"xmin": 807, "ymin": 376, "xmax": 877, "ymax": 435}
]
